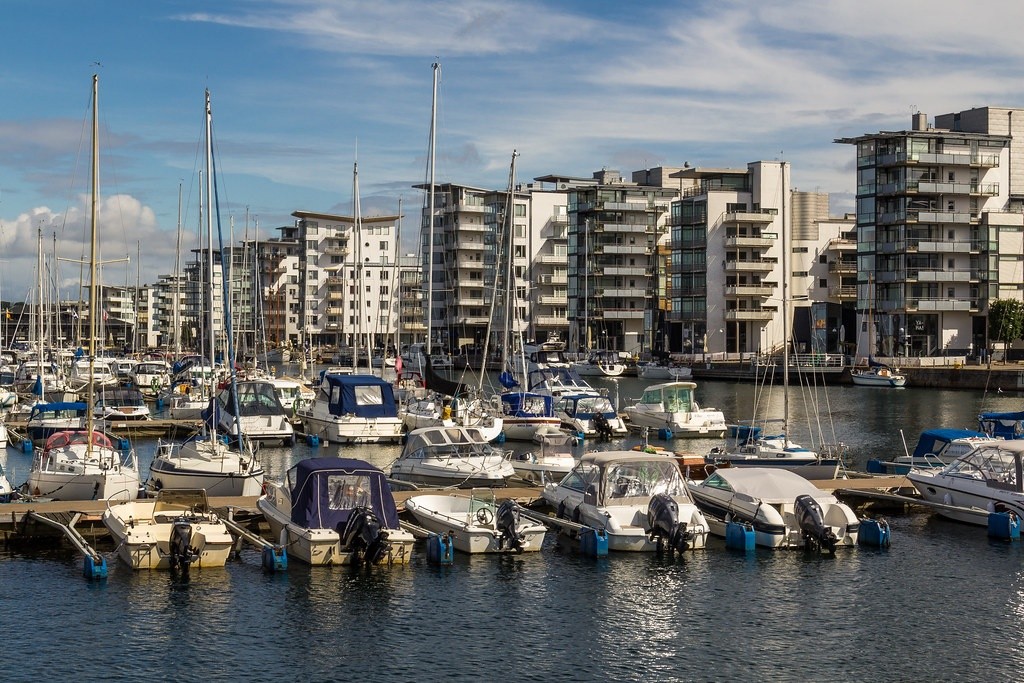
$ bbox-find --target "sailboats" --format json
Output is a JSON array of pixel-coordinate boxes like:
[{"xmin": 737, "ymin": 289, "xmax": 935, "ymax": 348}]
[{"xmin": 706, "ymin": 160, "xmax": 847, "ymax": 479}]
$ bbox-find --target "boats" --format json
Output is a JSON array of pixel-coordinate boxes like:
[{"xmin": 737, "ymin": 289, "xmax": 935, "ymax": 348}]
[
  {"xmin": 544, "ymin": 450, "xmax": 707, "ymax": 561},
  {"xmin": 862, "ymin": 408, "xmax": 1024, "ymax": 479},
  {"xmin": 513, "ymin": 449, "xmax": 619, "ymax": 488},
  {"xmin": 405, "ymin": 489, "xmax": 548, "ymax": 554},
  {"xmin": 909, "ymin": 441, "xmax": 1023, "ymax": 538},
  {"xmin": 684, "ymin": 466, "xmax": 860, "ymax": 555},
  {"xmin": 383, "ymin": 425, "xmax": 509, "ymax": 485},
  {"xmin": 33, "ymin": 428, "xmax": 143, "ymax": 502},
  {"xmin": 150, "ymin": 435, "xmax": 264, "ymax": 495},
  {"xmin": 1, "ymin": 62, "xmax": 726, "ymax": 449},
  {"xmin": 254, "ymin": 456, "xmax": 417, "ymax": 569},
  {"xmin": 850, "ymin": 356, "xmax": 906, "ymax": 388},
  {"xmin": 102, "ymin": 484, "xmax": 232, "ymax": 571}
]
